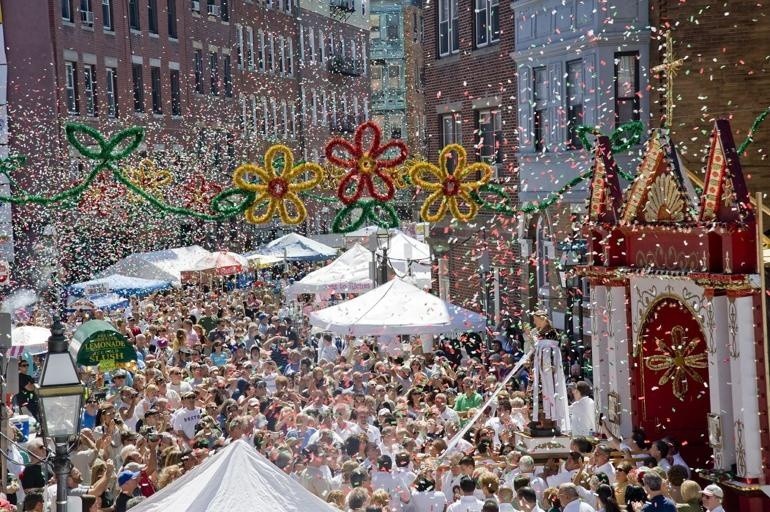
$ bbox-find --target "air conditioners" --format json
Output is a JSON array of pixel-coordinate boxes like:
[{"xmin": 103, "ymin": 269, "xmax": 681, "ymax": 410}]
[
  {"xmin": 208, "ymin": 5, "xmax": 218, "ymax": 16},
  {"xmin": 331, "ymin": 1, "xmax": 346, "ymax": 8},
  {"xmin": 191, "ymin": 1, "xmax": 200, "ymax": 11},
  {"xmin": 344, "ymin": 1, "xmax": 355, "ymax": 12}
]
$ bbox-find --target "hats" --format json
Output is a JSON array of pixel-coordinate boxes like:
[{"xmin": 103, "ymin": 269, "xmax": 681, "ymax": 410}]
[
  {"xmin": 117, "ymin": 470, "xmax": 141, "ymax": 485},
  {"xmin": 248, "ymin": 398, "xmax": 260, "ymax": 406},
  {"xmin": 124, "ymin": 462, "xmax": 148, "ymax": 472},
  {"xmin": 699, "ymin": 483, "xmax": 727, "ymax": 497}
]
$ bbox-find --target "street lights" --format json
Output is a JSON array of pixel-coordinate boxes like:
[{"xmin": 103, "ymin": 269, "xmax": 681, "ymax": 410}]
[
  {"xmin": 30, "ymin": 312, "xmax": 87, "ymax": 511},
  {"xmin": 375, "ymin": 227, "xmax": 395, "ymax": 283},
  {"xmin": 557, "ymin": 240, "xmax": 580, "ymax": 347}
]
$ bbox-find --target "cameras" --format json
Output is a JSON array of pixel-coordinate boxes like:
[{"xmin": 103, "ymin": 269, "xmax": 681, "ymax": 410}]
[{"xmin": 114, "ymin": 418, "xmax": 122, "ymax": 424}]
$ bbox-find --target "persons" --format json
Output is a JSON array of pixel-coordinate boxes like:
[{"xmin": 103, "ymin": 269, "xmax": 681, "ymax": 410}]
[{"xmin": 1, "ymin": 260, "xmax": 730, "ymax": 511}]
